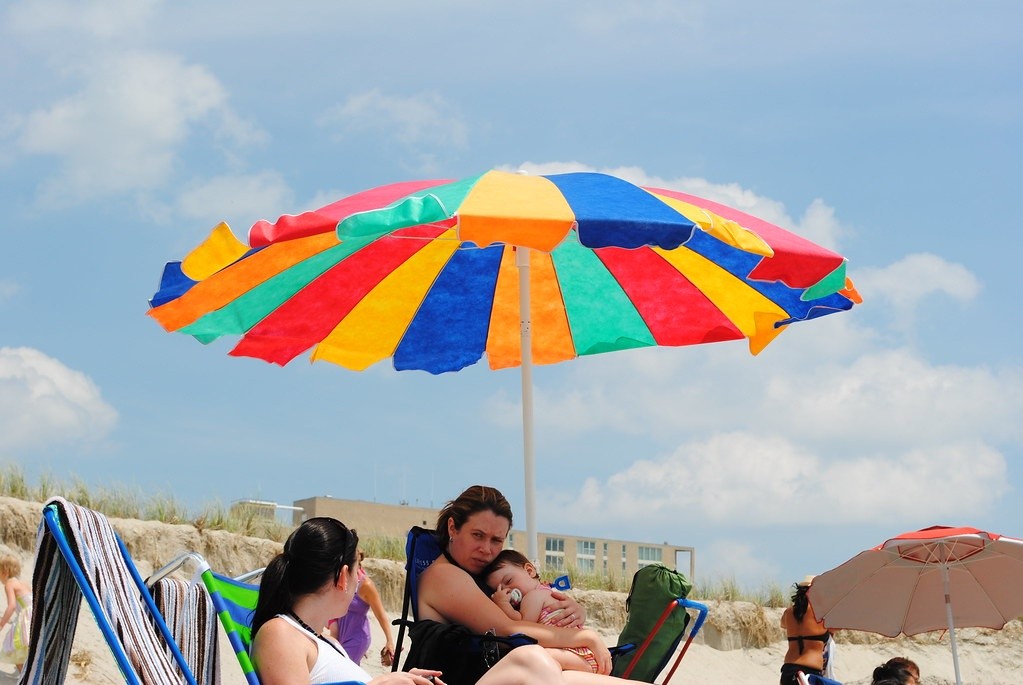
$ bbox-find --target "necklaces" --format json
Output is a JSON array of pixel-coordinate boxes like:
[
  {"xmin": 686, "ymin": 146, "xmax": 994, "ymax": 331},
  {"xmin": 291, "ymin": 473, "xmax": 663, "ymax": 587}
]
[{"xmin": 289, "ymin": 609, "xmax": 345, "ymax": 657}]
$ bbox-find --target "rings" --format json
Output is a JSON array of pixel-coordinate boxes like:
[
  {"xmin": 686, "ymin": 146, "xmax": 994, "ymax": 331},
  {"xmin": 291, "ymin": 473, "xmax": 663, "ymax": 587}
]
[{"xmin": 572, "ymin": 615, "xmax": 576, "ymax": 620}]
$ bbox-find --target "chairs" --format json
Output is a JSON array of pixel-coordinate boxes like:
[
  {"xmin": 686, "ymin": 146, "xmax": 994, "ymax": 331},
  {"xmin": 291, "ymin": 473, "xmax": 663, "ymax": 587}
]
[
  {"xmin": 17, "ymin": 496, "xmax": 198, "ymax": 685},
  {"xmin": 142, "ymin": 553, "xmax": 364, "ymax": 684},
  {"xmin": 392, "ymin": 524, "xmax": 635, "ymax": 685}
]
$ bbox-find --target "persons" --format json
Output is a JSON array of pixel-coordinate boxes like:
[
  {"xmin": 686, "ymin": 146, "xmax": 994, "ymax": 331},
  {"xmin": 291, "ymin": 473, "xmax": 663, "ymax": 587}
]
[
  {"xmin": 405, "ymin": 484, "xmax": 656, "ymax": 685},
  {"xmin": 873, "ymin": 654, "xmax": 920, "ymax": 685},
  {"xmin": 244, "ymin": 513, "xmax": 444, "ymax": 685},
  {"xmin": 323, "ymin": 549, "xmax": 395, "ymax": 665},
  {"xmin": 779, "ymin": 575, "xmax": 836, "ymax": 685}
]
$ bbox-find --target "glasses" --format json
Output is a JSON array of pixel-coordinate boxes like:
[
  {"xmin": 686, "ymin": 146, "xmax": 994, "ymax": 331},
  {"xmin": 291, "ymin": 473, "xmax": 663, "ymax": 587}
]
[
  {"xmin": 335, "ymin": 529, "xmax": 358, "ymax": 587},
  {"xmin": 484, "ymin": 628, "xmax": 500, "ymax": 669}
]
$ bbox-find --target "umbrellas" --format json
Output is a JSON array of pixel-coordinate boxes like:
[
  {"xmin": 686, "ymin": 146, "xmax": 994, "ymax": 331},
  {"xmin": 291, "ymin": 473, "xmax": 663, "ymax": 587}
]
[
  {"xmin": 806, "ymin": 525, "xmax": 1023, "ymax": 685},
  {"xmin": 147, "ymin": 168, "xmax": 862, "ymax": 575}
]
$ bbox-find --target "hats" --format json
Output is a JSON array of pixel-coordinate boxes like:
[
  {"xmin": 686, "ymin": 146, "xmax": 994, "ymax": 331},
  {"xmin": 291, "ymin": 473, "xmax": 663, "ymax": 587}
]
[{"xmin": 797, "ymin": 575, "xmax": 817, "ymax": 588}]
